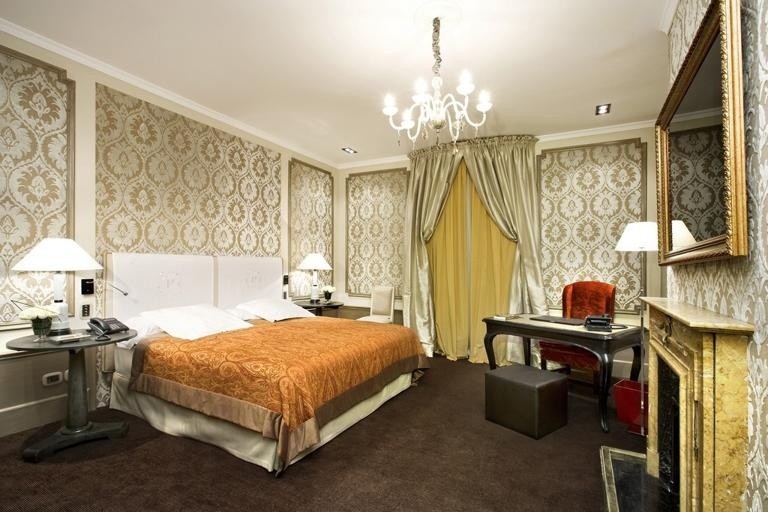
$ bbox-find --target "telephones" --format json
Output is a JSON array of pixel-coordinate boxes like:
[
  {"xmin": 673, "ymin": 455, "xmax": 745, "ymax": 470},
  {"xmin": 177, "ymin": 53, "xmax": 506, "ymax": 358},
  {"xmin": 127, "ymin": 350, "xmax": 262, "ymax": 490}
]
[{"xmin": 88, "ymin": 317, "xmax": 131, "ymax": 342}]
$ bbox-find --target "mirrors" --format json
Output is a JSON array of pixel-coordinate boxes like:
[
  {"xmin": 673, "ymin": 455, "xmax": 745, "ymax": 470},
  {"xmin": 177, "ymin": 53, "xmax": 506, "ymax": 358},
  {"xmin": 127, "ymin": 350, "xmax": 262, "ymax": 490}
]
[{"xmin": 653, "ymin": 2, "xmax": 749, "ymax": 266}]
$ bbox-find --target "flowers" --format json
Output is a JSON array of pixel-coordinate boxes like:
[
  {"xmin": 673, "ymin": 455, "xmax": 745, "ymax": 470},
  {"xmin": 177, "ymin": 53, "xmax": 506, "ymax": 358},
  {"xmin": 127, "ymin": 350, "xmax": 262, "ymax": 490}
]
[
  {"xmin": 321, "ymin": 286, "xmax": 337, "ymax": 292},
  {"xmin": 18, "ymin": 307, "xmax": 60, "ymax": 318}
]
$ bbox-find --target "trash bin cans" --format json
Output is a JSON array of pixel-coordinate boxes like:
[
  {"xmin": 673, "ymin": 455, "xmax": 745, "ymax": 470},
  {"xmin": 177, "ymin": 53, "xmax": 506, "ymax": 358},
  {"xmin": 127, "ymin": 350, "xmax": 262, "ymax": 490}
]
[{"xmin": 614, "ymin": 379, "xmax": 648, "ymax": 423}]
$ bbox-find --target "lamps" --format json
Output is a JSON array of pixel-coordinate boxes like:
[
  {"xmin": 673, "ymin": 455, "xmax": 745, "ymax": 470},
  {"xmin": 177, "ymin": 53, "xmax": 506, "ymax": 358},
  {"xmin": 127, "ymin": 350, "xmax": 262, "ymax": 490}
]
[
  {"xmin": 616, "ymin": 223, "xmax": 668, "ymax": 438},
  {"xmin": 298, "ymin": 254, "xmax": 333, "ymax": 304},
  {"xmin": 383, "ymin": 1, "xmax": 492, "ymax": 152},
  {"xmin": 12, "ymin": 238, "xmax": 104, "ymax": 343},
  {"xmin": 670, "ymin": 220, "xmax": 697, "ymax": 252}
]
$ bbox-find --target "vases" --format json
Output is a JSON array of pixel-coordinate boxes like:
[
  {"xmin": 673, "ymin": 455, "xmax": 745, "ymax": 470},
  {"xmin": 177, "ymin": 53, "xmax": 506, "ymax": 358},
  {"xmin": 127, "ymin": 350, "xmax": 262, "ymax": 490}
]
[
  {"xmin": 324, "ymin": 292, "xmax": 331, "ymax": 300},
  {"xmin": 28, "ymin": 318, "xmax": 52, "ymax": 338}
]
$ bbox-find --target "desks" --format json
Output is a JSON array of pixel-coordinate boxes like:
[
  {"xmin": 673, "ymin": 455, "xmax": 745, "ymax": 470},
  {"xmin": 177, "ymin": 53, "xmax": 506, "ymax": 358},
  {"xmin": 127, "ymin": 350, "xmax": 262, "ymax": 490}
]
[
  {"xmin": 5, "ymin": 326, "xmax": 138, "ymax": 463},
  {"xmin": 294, "ymin": 301, "xmax": 344, "ymax": 316}
]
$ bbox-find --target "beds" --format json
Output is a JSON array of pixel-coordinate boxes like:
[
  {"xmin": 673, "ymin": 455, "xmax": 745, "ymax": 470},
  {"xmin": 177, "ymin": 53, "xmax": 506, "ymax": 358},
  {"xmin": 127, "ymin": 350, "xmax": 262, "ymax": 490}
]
[{"xmin": 101, "ymin": 255, "xmax": 432, "ymax": 479}]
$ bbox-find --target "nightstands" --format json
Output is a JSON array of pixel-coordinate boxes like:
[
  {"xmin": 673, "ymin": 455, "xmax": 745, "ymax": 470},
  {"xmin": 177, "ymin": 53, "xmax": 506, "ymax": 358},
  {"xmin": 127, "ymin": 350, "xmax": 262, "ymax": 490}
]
[
  {"xmin": 292, "ymin": 295, "xmax": 311, "ymax": 305},
  {"xmin": 0, "ymin": 339, "xmax": 97, "ymax": 437}
]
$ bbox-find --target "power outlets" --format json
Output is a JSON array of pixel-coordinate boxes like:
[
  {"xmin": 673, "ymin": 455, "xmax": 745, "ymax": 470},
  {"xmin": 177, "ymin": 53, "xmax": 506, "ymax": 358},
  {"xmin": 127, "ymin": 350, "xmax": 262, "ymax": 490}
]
[{"xmin": 43, "ymin": 371, "xmax": 63, "ymax": 385}]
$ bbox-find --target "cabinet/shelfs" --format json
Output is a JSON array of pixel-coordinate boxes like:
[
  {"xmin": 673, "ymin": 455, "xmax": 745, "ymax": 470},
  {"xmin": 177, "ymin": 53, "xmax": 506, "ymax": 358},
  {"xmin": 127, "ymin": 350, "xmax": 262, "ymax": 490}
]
[{"xmin": 640, "ymin": 298, "xmax": 756, "ymax": 512}]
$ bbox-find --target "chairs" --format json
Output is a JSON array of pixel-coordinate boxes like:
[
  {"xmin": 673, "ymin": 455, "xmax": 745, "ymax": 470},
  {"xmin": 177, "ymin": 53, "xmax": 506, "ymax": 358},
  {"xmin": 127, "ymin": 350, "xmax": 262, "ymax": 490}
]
[{"xmin": 540, "ymin": 280, "xmax": 617, "ymax": 398}]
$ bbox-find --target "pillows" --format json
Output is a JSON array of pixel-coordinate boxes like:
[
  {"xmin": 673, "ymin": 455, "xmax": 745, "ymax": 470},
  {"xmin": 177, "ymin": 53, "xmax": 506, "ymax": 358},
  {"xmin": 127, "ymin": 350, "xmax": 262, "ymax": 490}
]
[
  {"xmin": 237, "ymin": 297, "xmax": 316, "ymax": 322},
  {"xmin": 138, "ymin": 303, "xmax": 255, "ymax": 340}
]
